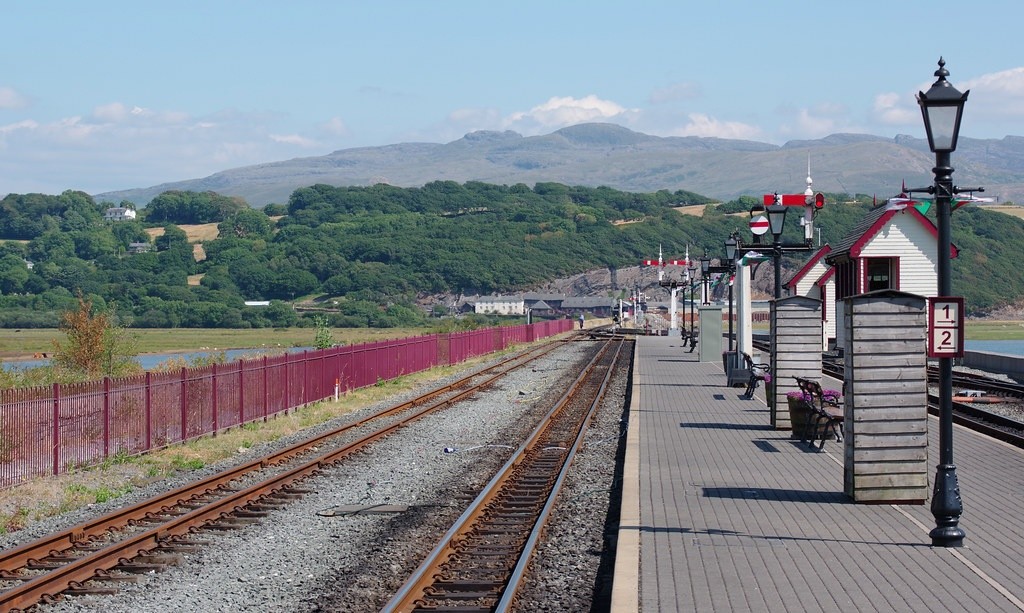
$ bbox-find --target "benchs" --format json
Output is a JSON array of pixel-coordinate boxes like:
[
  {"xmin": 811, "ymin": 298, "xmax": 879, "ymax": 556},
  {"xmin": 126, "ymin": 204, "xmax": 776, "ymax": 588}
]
[
  {"xmin": 739, "ymin": 351, "xmax": 771, "ymax": 399},
  {"xmin": 789, "ymin": 375, "xmax": 844, "ymax": 453},
  {"xmin": 681, "ymin": 327, "xmax": 698, "ymax": 353}
]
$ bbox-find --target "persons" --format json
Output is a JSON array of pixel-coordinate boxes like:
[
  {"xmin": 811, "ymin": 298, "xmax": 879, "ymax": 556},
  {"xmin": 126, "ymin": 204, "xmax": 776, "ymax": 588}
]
[
  {"xmin": 579, "ymin": 313, "xmax": 585, "ymax": 329},
  {"xmin": 613, "ymin": 315, "xmax": 622, "ymax": 328}
]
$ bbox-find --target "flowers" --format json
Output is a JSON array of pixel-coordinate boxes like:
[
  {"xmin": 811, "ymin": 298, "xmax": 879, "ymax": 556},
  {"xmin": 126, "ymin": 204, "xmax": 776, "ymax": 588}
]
[
  {"xmin": 787, "ymin": 390, "xmax": 841, "ymax": 401},
  {"xmin": 723, "ymin": 351, "xmax": 727, "ymax": 354},
  {"xmin": 763, "ymin": 375, "xmax": 771, "ymax": 382}
]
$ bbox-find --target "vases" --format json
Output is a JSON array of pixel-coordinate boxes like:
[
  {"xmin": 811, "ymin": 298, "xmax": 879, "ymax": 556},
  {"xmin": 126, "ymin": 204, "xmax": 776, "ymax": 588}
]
[
  {"xmin": 789, "ymin": 401, "xmax": 838, "ymax": 439},
  {"xmin": 765, "ymin": 383, "xmax": 770, "ymax": 407},
  {"xmin": 722, "ymin": 354, "xmax": 727, "ymax": 375}
]
